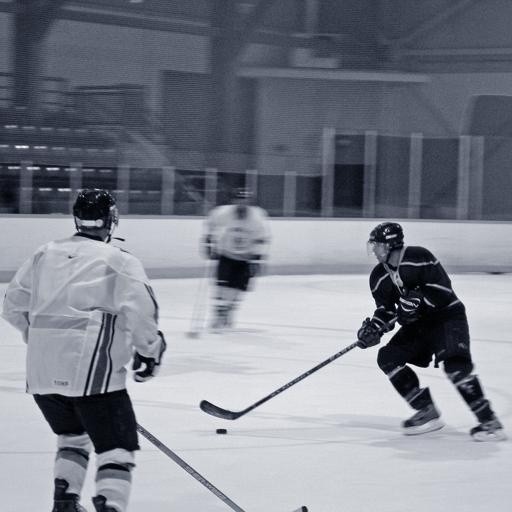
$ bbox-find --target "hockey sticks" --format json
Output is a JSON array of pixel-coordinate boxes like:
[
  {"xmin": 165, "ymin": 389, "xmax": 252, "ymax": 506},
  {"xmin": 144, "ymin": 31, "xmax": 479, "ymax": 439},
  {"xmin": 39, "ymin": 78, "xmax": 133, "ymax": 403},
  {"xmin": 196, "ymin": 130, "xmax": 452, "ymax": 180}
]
[
  {"xmin": 137, "ymin": 423, "xmax": 311, "ymax": 512},
  {"xmin": 199, "ymin": 339, "xmax": 358, "ymax": 422}
]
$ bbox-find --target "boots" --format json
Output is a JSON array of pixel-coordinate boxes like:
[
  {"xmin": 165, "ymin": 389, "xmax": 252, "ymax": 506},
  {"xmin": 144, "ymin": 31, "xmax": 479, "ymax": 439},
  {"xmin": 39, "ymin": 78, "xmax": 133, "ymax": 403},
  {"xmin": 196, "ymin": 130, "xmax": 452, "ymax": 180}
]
[
  {"xmin": 405, "ymin": 388, "xmax": 438, "ymax": 426},
  {"xmin": 471, "ymin": 401, "xmax": 502, "ymax": 433},
  {"xmin": 92, "ymin": 494, "xmax": 119, "ymax": 512},
  {"xmin": 50, "ymin": 478, "xmax": 86, "ymax": 512}
]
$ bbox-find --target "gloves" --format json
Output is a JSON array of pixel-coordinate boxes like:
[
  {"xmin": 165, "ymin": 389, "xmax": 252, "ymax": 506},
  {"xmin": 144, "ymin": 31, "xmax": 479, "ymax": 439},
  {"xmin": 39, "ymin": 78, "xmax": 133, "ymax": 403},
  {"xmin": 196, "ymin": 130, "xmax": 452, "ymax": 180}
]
[
  {"xmin": 396, "ymin": 297, "xmax": 421, "ymax": 323},
  {"xmin": 133, "ymin": 345, "xmax": 166, "ymax": 383},
  {"xmin": 359, "ymin": 317, "xmax": 383, "ymax": 346}
]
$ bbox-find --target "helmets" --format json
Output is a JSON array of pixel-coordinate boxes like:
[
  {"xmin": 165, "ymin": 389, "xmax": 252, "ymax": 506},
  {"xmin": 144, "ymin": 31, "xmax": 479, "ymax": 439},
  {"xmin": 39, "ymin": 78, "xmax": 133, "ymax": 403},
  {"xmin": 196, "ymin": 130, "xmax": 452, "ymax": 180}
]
[
  {"xmin": 366, "ymin": 224, "xmax": 403, "ymax": 253},
  {"xmin": 73, "ymin": 188, "xmax": 119, "ymax": 241},
  {"xmin": 232, "ymin": 187, "xmax": 249, "ymax": 198}
]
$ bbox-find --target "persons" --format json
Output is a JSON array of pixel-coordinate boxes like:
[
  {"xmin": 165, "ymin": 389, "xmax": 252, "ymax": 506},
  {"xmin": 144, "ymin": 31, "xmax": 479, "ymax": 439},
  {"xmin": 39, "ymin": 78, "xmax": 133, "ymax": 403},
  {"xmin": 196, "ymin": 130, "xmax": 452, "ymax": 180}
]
[
  {"xmin": 357, "ymin": 223, "xmax": 504, "ymax": 436},
  {"xmin": 0, "ymin": 188, "xmax": 167, "ymax": 512},
  {"xmin": 200, "ymin": 188, "xmax": 272, "ymax": 328}
]
